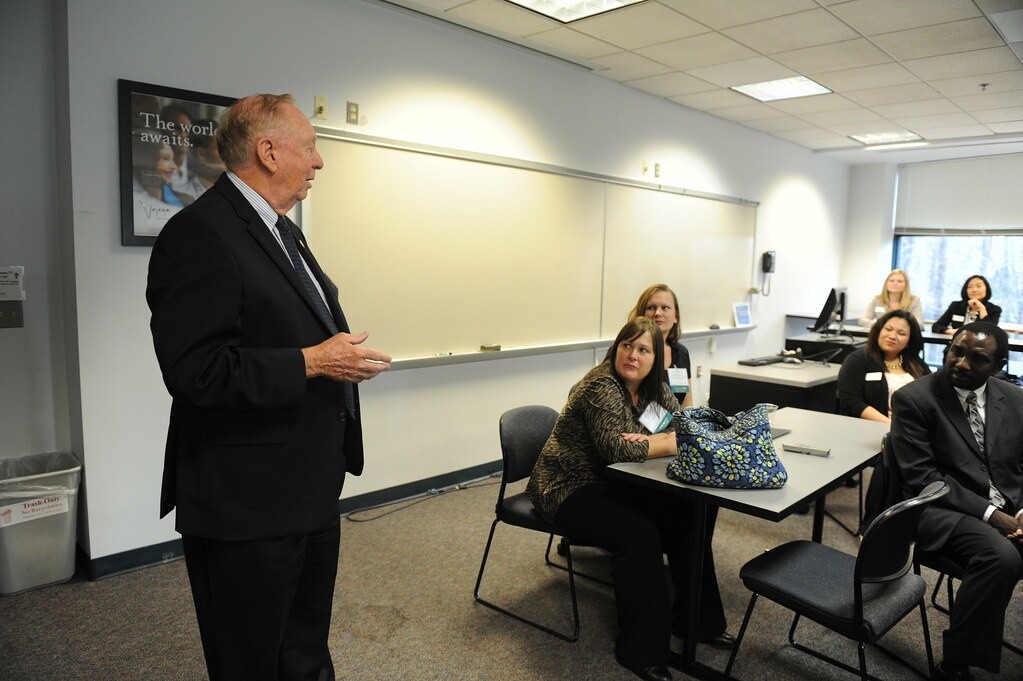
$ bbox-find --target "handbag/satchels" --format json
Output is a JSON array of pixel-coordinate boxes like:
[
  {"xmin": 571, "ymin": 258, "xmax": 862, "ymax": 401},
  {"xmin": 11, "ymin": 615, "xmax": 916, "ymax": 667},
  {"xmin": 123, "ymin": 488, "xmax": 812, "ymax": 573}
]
[
  {"xmin": 665, "ymin": 403, "xmax": 787, "ymax": 488},
  {"xmin": 859, "ymin": 433, "xmax": 894, "ymax": 535}
]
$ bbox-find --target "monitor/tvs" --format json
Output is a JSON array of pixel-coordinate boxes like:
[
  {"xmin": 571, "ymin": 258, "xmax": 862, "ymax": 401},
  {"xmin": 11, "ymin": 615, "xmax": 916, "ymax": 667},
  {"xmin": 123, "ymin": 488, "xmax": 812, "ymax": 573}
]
[{"xmin": 814, "ymin": 288, "xmax": 848, "ymax": 341}]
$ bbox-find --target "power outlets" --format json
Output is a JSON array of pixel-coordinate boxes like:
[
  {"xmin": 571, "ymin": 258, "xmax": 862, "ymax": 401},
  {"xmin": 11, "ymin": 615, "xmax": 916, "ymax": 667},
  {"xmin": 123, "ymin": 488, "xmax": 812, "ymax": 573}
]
[
  {"xmin": 655, "ymin": 164, "xmax": 660, "ymax": 178},
  {"xmin": 697, "ymin": 365, "xmax": 702, "ymax": 378},
  {"xmin": 346, "ymin": 100, "xmax": 359, "ymax": 125}
]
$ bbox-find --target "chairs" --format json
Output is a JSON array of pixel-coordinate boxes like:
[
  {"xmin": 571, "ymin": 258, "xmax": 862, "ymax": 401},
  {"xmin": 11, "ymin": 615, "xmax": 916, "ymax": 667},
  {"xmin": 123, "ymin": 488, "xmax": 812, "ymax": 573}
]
[
  {"xmin": 881, "ymin": 433, "xmax": 1023, "ymax": 681},
  {"xmin": 825, "ymin": 471, "xmax": 863, "ymax": 537},
  {"xmin": 473, "ymin": 405, "xmax": 619, "ymax": 643},
  {"xmin": 720, "ymin": 481, "xmax": 951, "ymax": 681}
]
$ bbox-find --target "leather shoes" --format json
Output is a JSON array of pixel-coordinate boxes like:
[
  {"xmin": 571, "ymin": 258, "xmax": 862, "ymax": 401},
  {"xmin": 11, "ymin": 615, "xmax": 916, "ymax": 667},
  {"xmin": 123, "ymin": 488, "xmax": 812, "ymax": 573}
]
[
  {"xmin": 934, "ymin": 660, "xmax": 975, "ymax": 681},
  {"xmin": 615, "ymin": 651, "xmax": 673, "ymax": 681},
  {"xmin": 672, "ymin": 624, "xmax": 737, "ymax": 648}
]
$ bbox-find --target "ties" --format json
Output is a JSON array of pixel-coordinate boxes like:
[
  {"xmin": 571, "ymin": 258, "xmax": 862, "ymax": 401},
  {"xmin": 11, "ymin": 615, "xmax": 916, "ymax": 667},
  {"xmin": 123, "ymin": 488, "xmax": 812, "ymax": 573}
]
[
  {"xmin": 275, "ymin": 215, "xmax": 359, "ymax": 421},
  {"xmin": 966, "ymin": 391, "xmax": 1007, "ymax": 509}
]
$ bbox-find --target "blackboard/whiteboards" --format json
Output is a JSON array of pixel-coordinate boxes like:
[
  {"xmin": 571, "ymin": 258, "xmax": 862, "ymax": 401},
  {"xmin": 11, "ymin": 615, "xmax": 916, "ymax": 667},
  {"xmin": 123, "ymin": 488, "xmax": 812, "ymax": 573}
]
[{"xmin": 302, "ymin": 121, "xmax": 761, "ymax": 372}]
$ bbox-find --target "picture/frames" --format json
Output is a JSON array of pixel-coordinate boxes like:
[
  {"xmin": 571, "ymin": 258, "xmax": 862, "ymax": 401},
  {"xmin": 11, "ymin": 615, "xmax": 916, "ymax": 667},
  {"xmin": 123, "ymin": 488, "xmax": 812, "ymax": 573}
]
[{"xmin": 116, "ymin": 77, "xmax": 245, "ymax": 248}]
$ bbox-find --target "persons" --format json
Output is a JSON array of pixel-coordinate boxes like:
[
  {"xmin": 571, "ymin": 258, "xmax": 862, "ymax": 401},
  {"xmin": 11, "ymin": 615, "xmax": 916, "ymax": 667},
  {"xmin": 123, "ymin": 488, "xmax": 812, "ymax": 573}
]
[
  {"xmin": 144, "ymin": 93, "xmax": 391, "ymax": 681},
  {"xmin": 628, "ymin": 284, "xmax": 691, "ymax": 417},
  {"xmin": 859, "ymin": 321, "xmax": 1023, "ymax": 681},
  {"xmin": 132, "ymin": 92, "xmax": 229, "ymax": 236},
  {"xmin": 857, "ymin": 269, "xmax": 925, "ymax": 329},
  {"xmin": 837, "ymin": 310, "xmax": 931, "ymax": 466},
  {"xmin": 527, "ymin": 317, "xmax": 736, "ymax": 681},
  {"xmin": 932, "ymin": 272, "xmax": 1002, "ymax": 336}
]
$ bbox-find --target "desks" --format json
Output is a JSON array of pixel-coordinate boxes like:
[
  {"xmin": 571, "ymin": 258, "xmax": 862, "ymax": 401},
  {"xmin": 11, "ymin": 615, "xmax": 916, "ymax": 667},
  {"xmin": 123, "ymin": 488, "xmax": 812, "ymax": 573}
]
[
  {"xmin": 784, "ymin": 315, "xmax": 1023, "ymax": 373},
  {"xmin": 707, "ymin": 360, "xmax": 844, "ymax": 419},
  {"xmin": 605, "ymin": 406, "xmax": 893, "ymax": 681}
]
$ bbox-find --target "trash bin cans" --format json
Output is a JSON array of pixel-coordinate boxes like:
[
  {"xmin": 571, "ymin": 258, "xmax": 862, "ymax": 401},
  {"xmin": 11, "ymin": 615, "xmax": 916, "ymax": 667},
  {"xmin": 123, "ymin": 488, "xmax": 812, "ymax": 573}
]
[{"xmin": 0, "ymin": 451, "xmax": 82, "ymax": 594}]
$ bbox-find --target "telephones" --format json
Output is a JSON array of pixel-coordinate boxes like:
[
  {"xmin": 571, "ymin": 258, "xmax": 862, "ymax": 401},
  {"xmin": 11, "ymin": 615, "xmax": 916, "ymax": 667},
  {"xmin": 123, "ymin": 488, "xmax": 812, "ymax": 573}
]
[{"xmin": 763, "ymin": 251, "xmax": 777, "ymax": 273}]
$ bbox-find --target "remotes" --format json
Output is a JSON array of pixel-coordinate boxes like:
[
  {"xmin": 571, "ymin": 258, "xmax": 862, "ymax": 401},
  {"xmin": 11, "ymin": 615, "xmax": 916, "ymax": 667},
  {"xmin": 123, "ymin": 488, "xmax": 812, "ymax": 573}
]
[{"xmin": 782, "ymin": 441, "xmax": 831, "ymax": 457}]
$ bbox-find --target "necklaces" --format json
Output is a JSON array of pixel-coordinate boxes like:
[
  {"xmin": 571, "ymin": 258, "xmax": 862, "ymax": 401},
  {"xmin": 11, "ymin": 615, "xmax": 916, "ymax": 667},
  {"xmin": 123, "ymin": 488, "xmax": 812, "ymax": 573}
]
[{"xmin": 884, "ymin": 355, "xmax": 903, "ymax": 370}]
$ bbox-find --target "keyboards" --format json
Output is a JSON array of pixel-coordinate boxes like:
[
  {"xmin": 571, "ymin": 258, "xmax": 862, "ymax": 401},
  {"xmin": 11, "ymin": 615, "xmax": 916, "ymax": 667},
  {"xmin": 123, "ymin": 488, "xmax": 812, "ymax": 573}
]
[{"xmin": 739, "ymin": 355, "xmax": 787, "ymax": 366}]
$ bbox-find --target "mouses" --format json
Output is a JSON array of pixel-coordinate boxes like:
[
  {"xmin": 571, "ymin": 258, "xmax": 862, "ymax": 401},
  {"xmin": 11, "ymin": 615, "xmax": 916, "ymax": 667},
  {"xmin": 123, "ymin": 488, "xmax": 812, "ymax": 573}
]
[{"xmin": 784, "ymin": 358, "xmax": 801, "ymax": 363}]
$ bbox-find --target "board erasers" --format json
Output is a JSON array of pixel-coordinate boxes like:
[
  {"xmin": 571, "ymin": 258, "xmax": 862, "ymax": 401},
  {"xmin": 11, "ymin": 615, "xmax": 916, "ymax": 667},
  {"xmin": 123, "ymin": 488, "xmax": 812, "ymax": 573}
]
[{"xmin": 480, "ymin": 344, "xmax": 501, "ymax": 350}]
